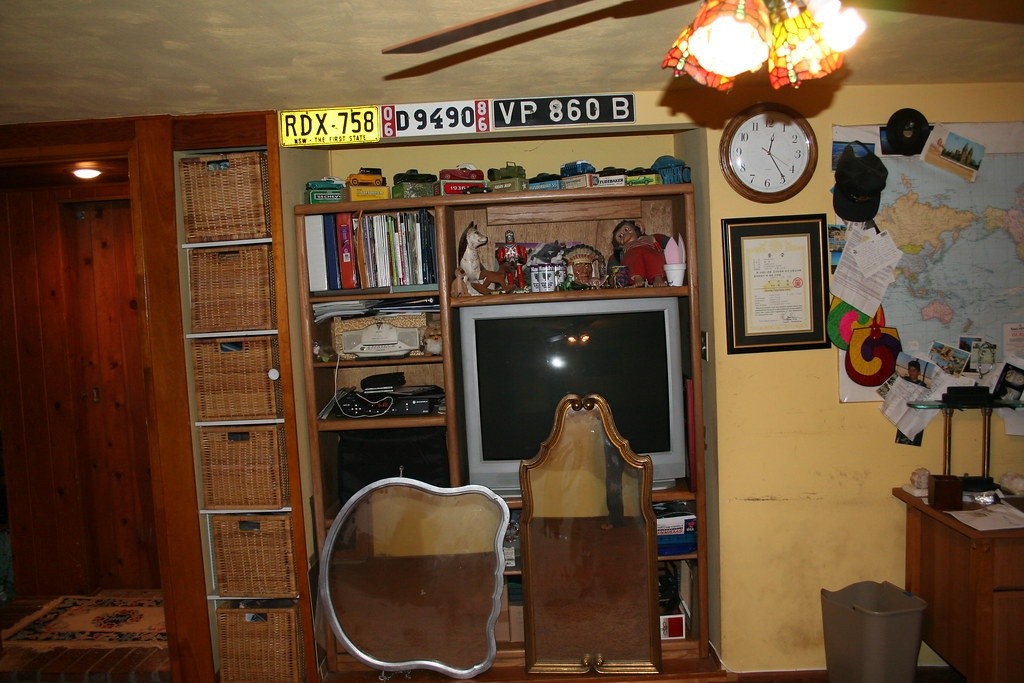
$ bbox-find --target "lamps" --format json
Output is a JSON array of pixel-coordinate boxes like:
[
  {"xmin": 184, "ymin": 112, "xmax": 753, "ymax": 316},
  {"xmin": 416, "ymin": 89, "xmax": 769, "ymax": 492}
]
[{"xmin": 661, "ymin": 0, "xmax": 870, "ymax": 93}]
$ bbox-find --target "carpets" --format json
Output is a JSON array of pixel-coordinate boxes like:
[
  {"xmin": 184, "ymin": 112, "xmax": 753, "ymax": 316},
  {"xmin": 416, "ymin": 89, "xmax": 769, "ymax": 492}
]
[{"xmin": 0, "ymin": 595, "xmax": 169, "ymax": 650}]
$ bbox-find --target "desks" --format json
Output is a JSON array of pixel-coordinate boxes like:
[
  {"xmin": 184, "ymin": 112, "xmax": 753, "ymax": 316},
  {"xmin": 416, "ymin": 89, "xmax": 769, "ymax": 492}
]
[{"xmin": 889, "ymin": 487, "xmax": 1024, "ymax": 683}]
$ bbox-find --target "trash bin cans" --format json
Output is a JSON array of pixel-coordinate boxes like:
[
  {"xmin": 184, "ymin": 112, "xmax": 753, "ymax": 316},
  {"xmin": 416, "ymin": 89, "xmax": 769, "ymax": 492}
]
[{"xmin": 821, "ymin": 580, "xmax": 928, "ymax": 683}]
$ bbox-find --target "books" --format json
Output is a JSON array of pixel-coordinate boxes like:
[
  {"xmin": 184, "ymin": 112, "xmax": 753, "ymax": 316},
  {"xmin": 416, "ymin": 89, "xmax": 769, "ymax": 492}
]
[
  {"xmin": 303, "ymin": 209, "xmax": 437, "ymax": 292},
  {"xmin": 311, "ymin": 298, "xmax": 441, "ymax": 324}
]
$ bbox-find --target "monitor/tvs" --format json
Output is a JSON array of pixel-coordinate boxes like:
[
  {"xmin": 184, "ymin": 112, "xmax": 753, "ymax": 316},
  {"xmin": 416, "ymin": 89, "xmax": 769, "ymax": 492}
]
[{"xmin": 459, "ymin": 298, "xmax": 687, "ymax": 498}]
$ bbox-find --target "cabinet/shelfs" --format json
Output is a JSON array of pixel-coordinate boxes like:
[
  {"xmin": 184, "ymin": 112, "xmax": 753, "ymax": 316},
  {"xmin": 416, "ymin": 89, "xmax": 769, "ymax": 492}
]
[{"xmin": 292, "ymin": 183, "xmax": 713, "ymax": 665}]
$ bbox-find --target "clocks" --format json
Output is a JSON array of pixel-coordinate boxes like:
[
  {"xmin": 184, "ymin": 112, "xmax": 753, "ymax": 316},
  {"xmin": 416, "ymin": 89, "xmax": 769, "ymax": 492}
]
[{"xmin": 720, "ymin": 103, "xmax": 815, "ymax": 203}]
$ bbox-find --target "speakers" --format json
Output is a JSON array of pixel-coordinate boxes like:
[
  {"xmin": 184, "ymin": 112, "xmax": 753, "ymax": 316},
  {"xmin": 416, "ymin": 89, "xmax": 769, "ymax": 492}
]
[{"xmin": 342, "ymin": 322, "xmax": 420, "ymax": 358}]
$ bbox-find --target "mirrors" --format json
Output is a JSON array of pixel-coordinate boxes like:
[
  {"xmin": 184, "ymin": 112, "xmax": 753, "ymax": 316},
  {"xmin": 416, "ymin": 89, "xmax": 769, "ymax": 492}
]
[
  {"xmin": 519, "ymin": 393, "xmax": 665, "ymax": 676},
  {"xmin": 320, "ymin": 475, "xmax": 511, "ymax": 682}
]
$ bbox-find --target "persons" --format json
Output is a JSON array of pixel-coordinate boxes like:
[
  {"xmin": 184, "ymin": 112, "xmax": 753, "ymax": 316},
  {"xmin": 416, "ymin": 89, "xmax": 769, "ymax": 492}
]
[{"xmin": 903, "ymin": 361, "xmax": 927, "ymax": 387}]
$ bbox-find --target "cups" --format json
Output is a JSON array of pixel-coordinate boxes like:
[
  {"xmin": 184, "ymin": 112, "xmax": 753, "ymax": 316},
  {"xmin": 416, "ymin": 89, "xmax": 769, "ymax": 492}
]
[{"xmin": 663, "ymin": 263, "xmax": 686, "ymax": 286}]
